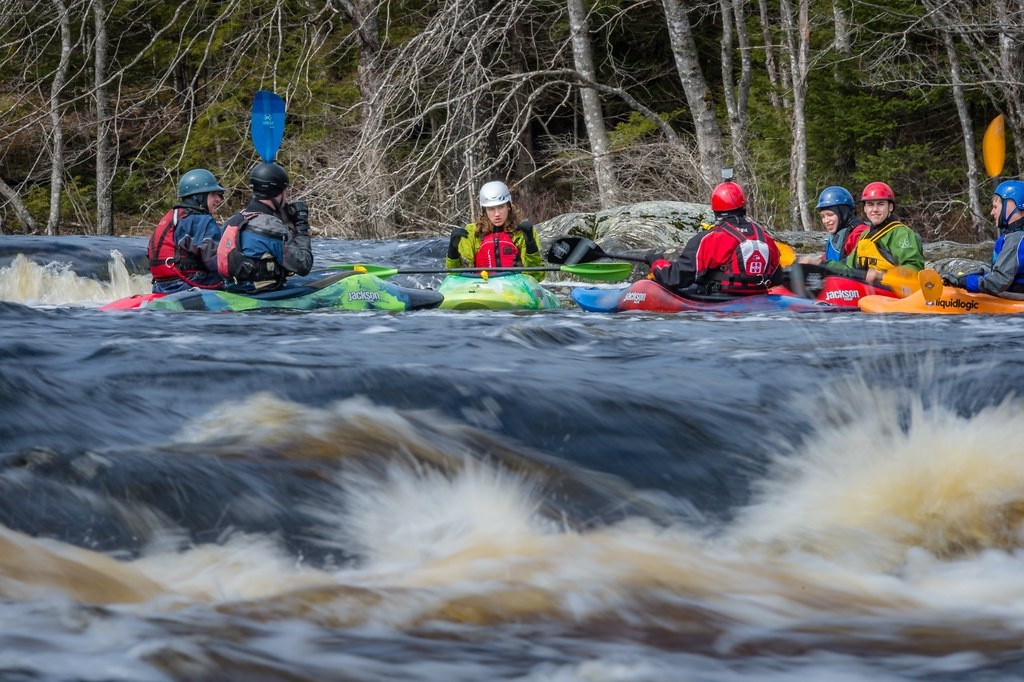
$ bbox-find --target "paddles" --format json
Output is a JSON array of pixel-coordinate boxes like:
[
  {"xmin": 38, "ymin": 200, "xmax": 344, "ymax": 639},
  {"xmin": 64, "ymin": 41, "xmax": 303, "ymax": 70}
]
[
  {"xmin": 981, "ymin": 112, "xmax": 1007, "ymax": 241},
  {"xmin": 772, "ymin": 239, "xmax": 920, "ymax": 298},
  {"xmin": 917, "ymin": 267, "xmax": 982, "ymax": 303},
  {"xmin": 547, "ymin": 236, "xmax": 648, "ymax": 264},
  {"xmin": 331, "ymin": 261, "xmax": 635, "ymax": 281},
  {"xmin": 248, "ymin": 89, "xmax": 288, "ymax": 165}
]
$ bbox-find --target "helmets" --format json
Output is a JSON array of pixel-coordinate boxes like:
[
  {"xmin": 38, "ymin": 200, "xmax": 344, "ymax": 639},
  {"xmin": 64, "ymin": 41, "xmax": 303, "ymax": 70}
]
[
  {"xmin": 711, "ymin": 181, "xmax": 745, "ymax": 211},
  {"xmin": 994, "ymin": 180, "xmax": 1024, "ymax": 210},
  {"xmin": 250, "ymin": 163, "xmax": 292, "ymax": 192},
  {"xmin": 178, "ymin": 169, "xmax": 225, "ymax": 197},
  {"xmin": 860, "ymin": 182, "xmax": 895, "ymax": 203},
  {"xmin": 814, "ymin": 186, "xmax": 856, "ymax": 209},
  {"xmin": 479, "ymin": 181, "xmax": 510, "ymax": 207}
]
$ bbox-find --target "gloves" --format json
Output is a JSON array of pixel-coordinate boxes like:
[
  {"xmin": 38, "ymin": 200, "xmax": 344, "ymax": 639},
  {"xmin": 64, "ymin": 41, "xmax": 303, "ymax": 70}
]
[
  {"xmin": 284, "ymin": 201, "xmax": 308, "ymax": 224},
  {"xmin": 448, "ymin": 228, "xmax": 469, "ymax": 258},
  {"xmin": 645, "ymin": 251, "xmax": 664, "ymax": 266},
  {"xmin": 941, "ymin": 273, "xmax": 958, "ymax": 286},
  {"xmin": 516, "ymin": 221, "xmax": 534, "ymax": 245}
]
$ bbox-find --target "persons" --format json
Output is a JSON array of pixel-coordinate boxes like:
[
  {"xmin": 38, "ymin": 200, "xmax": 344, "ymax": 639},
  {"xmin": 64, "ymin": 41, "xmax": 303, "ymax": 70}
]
[
  {"xmin": 220, "ymin": 160, "xmax": 313, "ymax": 295},
  {"xmin": 941, "ymin": 180, "xmax": 1024, "ymax": 303},
  {"xmin": 146, "ymin": 168, "xmax": 227, "ymax": 296},
  {"xmin": 445, "ymin": 181, "xmax": 546, "ymax": 283},
  {"xmin": 644, "ymin": 181, "xmax": 779, "ymax": 296},
  {"xmin": 800, "ymin": 184, "xmax": 873, "ymax": 277},
  {"xmin": 798, "ymin": 181, "xmax": 925, "ymax": 285}
]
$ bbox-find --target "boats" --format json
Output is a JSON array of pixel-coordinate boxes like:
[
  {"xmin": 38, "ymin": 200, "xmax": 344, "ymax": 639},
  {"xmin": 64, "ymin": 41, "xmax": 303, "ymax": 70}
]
[
  {"xmin": 98, "ymin": 270, "xmax": 444, "ymax": 313},
  {"xmin": 570, "ymin": 279, "xmax": 1024, "ymax": 318},
  {"xmin": 438, "ymin": 272, "xmax": 562, "ymax": 311}
]
